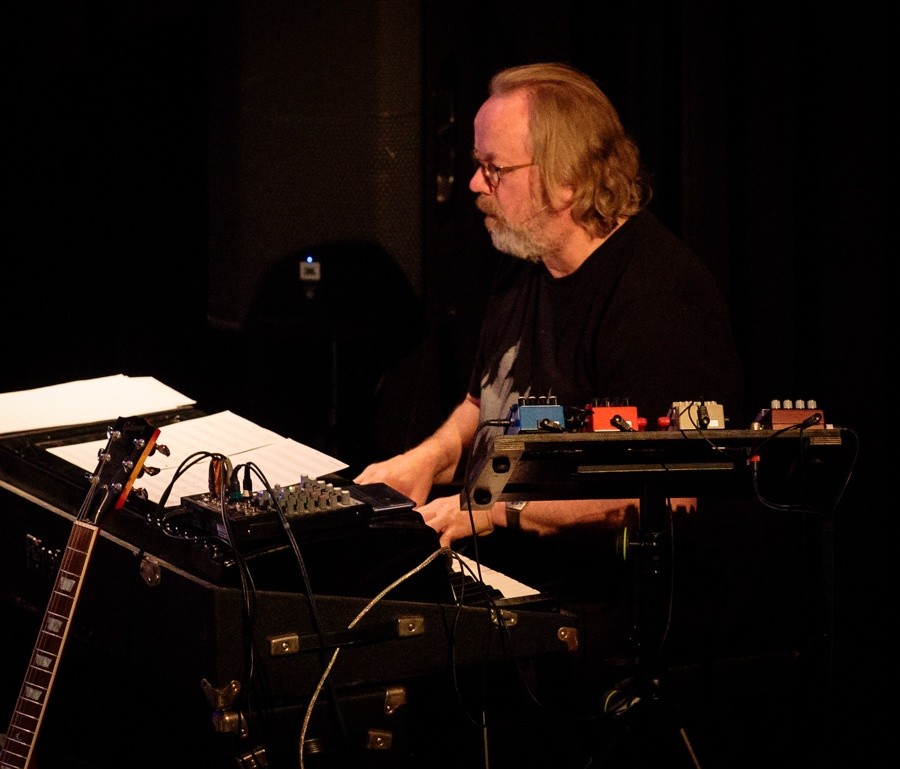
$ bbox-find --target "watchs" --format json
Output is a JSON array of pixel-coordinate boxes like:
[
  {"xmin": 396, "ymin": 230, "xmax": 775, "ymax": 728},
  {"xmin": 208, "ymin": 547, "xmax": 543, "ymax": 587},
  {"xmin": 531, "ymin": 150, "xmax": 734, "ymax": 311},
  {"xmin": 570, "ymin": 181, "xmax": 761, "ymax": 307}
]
[{"xmin": 505, "ymin": 502, "xmax": 528, "ymax": 532}]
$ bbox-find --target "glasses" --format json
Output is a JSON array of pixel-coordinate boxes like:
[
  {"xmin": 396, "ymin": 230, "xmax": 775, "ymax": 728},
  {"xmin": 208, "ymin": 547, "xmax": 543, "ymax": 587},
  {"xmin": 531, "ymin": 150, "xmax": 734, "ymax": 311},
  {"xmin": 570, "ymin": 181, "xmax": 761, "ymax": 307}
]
[{"xmin": 471, "ymin": 157, "xmax": 538, "ymax": 187}]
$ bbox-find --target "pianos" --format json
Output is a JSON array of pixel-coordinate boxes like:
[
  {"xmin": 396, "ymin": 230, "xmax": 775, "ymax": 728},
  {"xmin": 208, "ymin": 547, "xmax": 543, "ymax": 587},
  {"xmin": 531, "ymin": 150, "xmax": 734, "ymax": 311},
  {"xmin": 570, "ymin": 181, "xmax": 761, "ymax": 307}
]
[{"xmin": 0, "ymin": 373, "xmax": 589, "ymax": 769}]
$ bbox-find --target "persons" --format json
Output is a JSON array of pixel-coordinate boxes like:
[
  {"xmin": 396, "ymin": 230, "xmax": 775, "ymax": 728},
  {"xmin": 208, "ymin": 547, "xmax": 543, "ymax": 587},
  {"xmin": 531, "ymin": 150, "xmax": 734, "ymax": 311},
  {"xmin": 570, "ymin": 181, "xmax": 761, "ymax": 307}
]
[{"xmin": 353, "ymin": 62, "xmax": 750, "ymax": 589}]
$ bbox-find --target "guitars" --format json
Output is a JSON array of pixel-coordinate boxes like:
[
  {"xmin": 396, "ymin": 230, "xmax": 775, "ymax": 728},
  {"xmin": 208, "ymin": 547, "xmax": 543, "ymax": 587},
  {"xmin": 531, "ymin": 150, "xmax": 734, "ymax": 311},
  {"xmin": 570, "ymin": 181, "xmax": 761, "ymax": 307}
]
[{"xmin": 0, "ymin": 416, "xmax": 171, "ymax": 769}]
